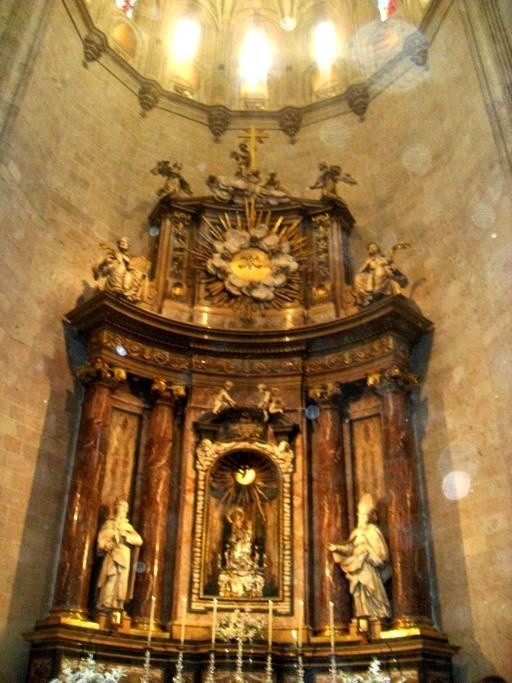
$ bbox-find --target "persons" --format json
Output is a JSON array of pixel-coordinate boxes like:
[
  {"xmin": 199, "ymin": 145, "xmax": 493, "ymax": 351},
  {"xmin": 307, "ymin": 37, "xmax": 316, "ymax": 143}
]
[
  {"xmin": 355, "ymin": 241, "xmax": 403, "ymax": 301},
  {"xmin": 157, "ymin": 143, "xmax": 357, "ymax": 199},
  {"xmin": 212, "ymin": 381, "xmax": 237, "ymax": 415},
  {"xmin": 97, "ymin": 236, "xmax": 147, "ymax": 301},
  {"xmin": 95, "ymin": 497, "xmax": 144, "ymax": 612},
  {"xmin": 256, "ymin": 383, "xmax": 284, "ymax": 422},
  {"xmin": 326, "ymin": 491, "xmax": 391, "ymax": 633}
]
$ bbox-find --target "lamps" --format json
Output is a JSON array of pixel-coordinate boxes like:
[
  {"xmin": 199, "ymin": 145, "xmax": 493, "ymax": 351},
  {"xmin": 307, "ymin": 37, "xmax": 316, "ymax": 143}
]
[
  {"xmin": 357, "ymin": 616, "xmax": 371, "ymax": 644},
  {"xmin": 109, "ymin": 608, "xmax": 122, "ymax": 637}
]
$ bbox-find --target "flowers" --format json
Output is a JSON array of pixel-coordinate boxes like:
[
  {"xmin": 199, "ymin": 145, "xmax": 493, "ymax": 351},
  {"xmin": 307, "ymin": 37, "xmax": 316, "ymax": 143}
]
[
  {"xmin": 49, "ymin": 651, "xmax": 128, "ymax": 683},
  {"xmin": 213, "ymin": 601, "xmax": 267, "ymax": 644},
  {"xmin": 335, "ymin": 656, "xmax": 408, "ymax": 683}
]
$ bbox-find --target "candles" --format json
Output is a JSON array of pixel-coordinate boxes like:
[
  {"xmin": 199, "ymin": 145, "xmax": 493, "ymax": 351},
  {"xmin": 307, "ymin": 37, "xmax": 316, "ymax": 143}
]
[
  {"xmin": 148, "ymin": 596, "xmax": 156, "ymax": 642},
  {"xmin": 211, "ymin": 597, "xmax": 218, "ymax": 644},
  {"xmin": 329, "ymin": 601, "xmax": 335, "ymax": 648},
  {"xmin": 268, "ymin": 600, "xmax": 273, "ymax": 646},
  {"xmin": 297, "ymin": 600, "xmax": 304, "ymax": 648},
  {"xmin": 181, "ymin": 598, "xmax": 188, "ymax": 644}
]
